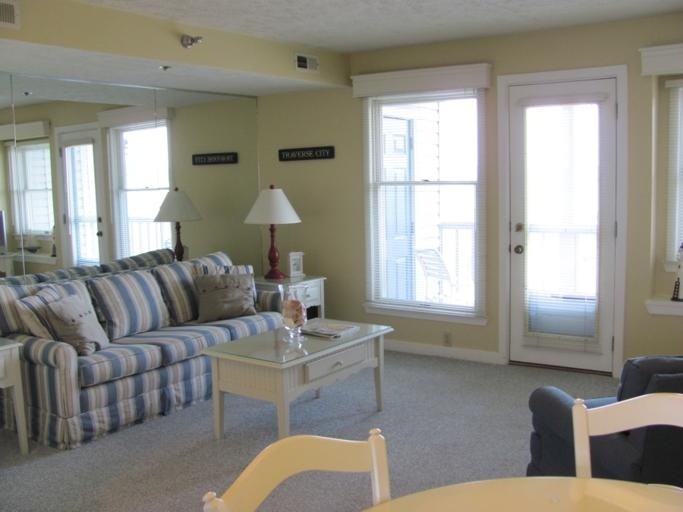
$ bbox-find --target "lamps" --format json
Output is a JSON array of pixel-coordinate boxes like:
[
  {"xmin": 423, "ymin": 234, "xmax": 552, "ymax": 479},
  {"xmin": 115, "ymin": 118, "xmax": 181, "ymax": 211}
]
[
  {"xmin": 154, "ymin": 186, "xmax": 201, "ymax": 261},
  {"xmin": 244, "ymin": 187, "xmax": 301, "ymax": 280}
]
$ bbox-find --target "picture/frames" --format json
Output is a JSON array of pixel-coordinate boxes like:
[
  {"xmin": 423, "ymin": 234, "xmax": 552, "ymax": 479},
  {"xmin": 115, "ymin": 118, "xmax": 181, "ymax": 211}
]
[{"xmin": 287, "ymin": 251, "xmax": 304, "ymax": 278}]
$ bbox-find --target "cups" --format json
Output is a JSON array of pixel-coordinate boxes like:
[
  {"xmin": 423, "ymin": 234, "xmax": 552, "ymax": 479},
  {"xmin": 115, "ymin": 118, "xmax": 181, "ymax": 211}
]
[{"xmin": 289, "ymin": 250, "xmax": 304, "ymax": 281}]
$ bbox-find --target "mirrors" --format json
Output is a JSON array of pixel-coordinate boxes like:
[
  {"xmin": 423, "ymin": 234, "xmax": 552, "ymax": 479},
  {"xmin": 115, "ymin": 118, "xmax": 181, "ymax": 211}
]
[{"xmin": 1, "ymin": 69, "xmax": 265, "ymax": 286}]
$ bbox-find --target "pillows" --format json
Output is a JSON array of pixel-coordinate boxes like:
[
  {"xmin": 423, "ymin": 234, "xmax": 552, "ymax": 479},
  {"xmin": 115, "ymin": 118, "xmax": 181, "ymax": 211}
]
[
  {"xmin": 45, "ymin": 295, "xmax": 109, "ymax": 356},
  {"xmin": 192, "ymin": 263, "xmax": 256, "ymax": 322}
]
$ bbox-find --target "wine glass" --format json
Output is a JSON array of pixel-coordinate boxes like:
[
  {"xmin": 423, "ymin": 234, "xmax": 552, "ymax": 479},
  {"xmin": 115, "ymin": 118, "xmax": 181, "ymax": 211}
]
[{"xmin": 278, "ymin": 285, "xmax": 310, "ymax": 344}]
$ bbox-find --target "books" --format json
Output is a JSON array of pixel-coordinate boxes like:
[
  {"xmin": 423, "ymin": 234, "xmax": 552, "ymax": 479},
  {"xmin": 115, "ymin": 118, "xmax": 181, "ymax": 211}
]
[{"xmin": 300, "ymin": 321, "xmax": 360, "ymax": 340}]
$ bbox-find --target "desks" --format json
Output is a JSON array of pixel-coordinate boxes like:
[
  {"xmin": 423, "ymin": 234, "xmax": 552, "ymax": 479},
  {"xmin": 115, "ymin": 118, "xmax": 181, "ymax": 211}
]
[
  {"xmin": 353, "ymin": 474, "xmax": 681, "ymax": 512},
  {"xmin": 254, "ymin": 273, "xmax": 326, "ymax": 322}
]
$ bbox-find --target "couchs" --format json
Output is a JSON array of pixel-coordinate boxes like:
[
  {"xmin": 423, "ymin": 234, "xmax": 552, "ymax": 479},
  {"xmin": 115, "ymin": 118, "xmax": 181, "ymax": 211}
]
[{"xmin": 1, "ymin": 250, "xmax": 283, "ymax": 452}]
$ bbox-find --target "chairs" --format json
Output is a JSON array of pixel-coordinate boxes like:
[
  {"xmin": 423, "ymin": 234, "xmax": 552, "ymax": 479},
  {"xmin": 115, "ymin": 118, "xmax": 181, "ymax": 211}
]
[
  {"xmin": 524, "ymin": 355, "xmax": 682, "ymax": 483},
  {"xmin": 202, "ymin": 429, "xmax": 392, "ymax": 512}
]
